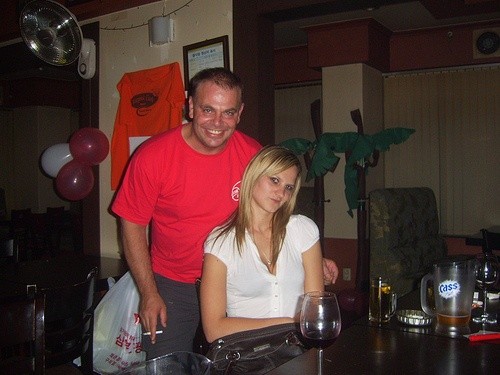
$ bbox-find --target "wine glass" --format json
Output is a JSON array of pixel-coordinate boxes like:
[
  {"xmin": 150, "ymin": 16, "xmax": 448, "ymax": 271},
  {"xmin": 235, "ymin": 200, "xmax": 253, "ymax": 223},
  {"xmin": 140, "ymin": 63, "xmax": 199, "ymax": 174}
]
[
  {"xmin": 299, "ymin": 290, "xmax": 341, "ymax": 375},
  {"xmin": 472, "ymin": 253, "xmax": 498, "ymax": 323}
]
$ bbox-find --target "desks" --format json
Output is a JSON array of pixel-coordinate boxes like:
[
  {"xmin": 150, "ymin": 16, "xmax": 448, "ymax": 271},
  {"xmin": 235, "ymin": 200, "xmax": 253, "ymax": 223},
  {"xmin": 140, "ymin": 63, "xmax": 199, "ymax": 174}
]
[
  {"xmin": 465, "ymin": 225, "xmax": 500, "ymax": 247},
  {"xmin": 30, "ymin": 213, "xmax": 83, "ymax": 244}
]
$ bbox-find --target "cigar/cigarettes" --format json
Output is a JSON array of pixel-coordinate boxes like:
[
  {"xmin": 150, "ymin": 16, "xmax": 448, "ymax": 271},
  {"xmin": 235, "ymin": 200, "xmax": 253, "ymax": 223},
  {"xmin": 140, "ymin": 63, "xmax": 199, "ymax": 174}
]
[{"xmin": 143, "ymin": 331, "xmax": 163, "ymax": 336}]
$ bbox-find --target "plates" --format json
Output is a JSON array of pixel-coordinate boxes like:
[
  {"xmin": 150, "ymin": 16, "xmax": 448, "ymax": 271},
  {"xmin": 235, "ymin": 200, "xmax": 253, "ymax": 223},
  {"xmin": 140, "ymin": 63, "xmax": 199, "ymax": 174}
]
[{"xmin": 395, "ymin": 310, "xmax": 432, "ymax": 326}]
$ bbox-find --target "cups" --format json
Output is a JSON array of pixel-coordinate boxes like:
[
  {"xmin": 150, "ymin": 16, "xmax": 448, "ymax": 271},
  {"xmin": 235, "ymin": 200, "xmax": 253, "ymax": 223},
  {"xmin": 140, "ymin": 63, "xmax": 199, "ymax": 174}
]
[{"xmin": 368, "ymin": 275, "xmax": 397, "ymax": 323}]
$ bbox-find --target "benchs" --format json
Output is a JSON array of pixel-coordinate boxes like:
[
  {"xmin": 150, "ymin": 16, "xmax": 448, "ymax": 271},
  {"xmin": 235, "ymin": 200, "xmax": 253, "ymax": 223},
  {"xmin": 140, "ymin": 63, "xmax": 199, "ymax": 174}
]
[{"xmin": 367, "ymin": 186, "xmax": 449, "ymax": 298}]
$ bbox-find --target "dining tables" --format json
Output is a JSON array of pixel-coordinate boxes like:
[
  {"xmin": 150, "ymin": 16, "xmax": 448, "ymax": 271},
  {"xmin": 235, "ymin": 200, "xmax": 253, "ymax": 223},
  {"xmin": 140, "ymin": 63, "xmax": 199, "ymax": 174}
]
[
  {"xmin": 263, "ymin": 325, "xmax": 500, "ymax": 375},
  {"xmin": 364, "ymin": 287, "xmax": 500, "ymax": 340}
]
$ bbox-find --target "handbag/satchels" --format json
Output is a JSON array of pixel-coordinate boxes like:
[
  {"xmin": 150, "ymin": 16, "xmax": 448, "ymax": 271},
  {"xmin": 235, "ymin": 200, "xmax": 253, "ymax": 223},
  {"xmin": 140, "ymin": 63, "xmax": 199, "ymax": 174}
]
[
  {"xmin": 91, "ymin": 273, "xmax": 147, "ymax": 374},
  {"xmin": 206, "ymin": 322, "xmax": 308, "ymax": 375}
]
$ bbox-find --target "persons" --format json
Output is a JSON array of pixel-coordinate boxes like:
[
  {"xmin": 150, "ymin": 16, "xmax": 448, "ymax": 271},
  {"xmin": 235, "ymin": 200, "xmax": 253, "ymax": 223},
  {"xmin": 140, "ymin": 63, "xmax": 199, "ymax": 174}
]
[
  {"xmin": 111, "ymin": 66, "xmax": 338, "ymax": 363},
  {"xmin": 200, "ymin": 146, "xmax": 324, "ymax": 343}
]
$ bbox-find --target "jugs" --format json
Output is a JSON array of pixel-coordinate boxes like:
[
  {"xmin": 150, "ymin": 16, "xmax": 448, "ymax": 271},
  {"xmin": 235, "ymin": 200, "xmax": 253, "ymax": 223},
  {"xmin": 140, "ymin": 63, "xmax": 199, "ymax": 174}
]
[{"xmin": 421, "ymin": 260, "xmax": 481, "ymax": 333}]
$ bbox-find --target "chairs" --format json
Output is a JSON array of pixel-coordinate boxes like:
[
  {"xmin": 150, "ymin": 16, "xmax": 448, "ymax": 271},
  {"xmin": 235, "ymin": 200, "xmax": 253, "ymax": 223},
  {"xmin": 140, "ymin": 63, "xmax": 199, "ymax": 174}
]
[
  {"xmin": 45, "ymin": 206, "xmax": 76, "ymax": 257},
  {"xmin": 480, "ymin": 227, "xmax": 500, "ymax": 257},
  {"xmin": 21, "ymin": 254, "xmax": 100, "ymax": 375},
  {"xmin": 7, "ymin": 207, "xmax": 37, "ymax": 266}
]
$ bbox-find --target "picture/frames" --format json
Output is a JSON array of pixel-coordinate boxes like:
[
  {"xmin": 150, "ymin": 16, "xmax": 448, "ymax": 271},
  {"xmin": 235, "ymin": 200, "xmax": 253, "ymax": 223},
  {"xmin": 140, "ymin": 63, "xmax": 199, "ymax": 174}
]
[{"xmin": 181, "ymin": 35, "xmax": 230, "ymax": 91}]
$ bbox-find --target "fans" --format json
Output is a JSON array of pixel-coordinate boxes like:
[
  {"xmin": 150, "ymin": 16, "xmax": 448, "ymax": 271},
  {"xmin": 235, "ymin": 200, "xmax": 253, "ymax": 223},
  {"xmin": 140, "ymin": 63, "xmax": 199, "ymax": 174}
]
[{"xmin": 18, "ymin": 0, "xmax": 97, "ymax": 80}]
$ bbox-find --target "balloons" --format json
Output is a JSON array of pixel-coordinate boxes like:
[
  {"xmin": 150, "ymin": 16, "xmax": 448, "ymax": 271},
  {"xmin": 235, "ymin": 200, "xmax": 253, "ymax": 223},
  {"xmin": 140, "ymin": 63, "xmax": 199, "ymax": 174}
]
[
  {"xmin": 40, "ymin": 143, "xmax": 63, "ymax": 173},
  {"xmin": 56, "ymin": 160, "xmax": 94, "ymax": 201},
  {"xmin": 69, "ymin": 128, "xmax": 109, "ymax": 165}
]
